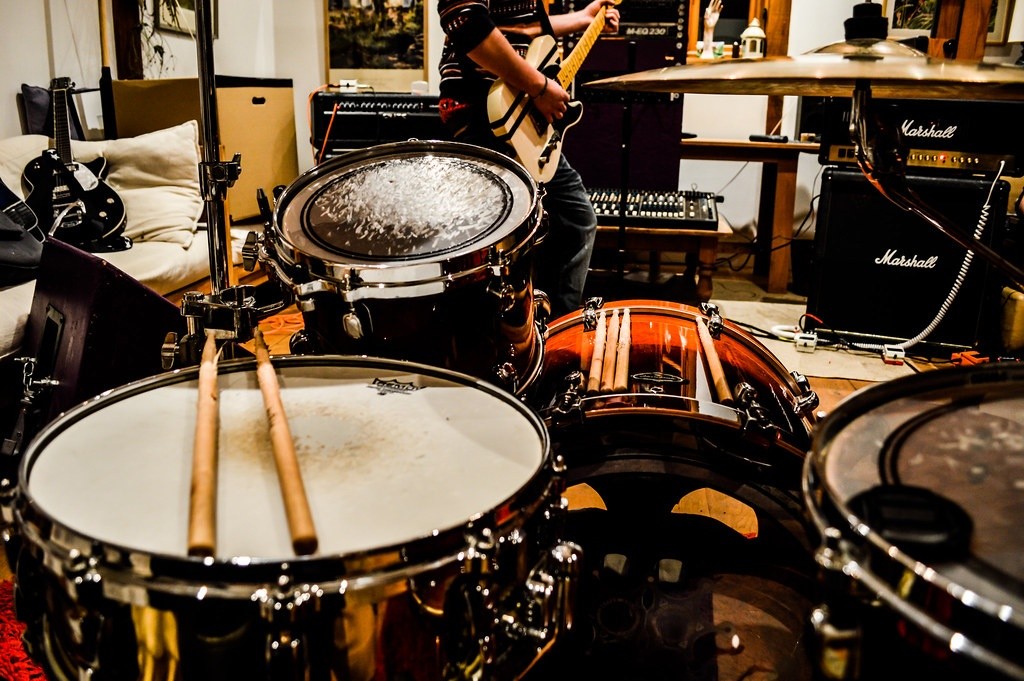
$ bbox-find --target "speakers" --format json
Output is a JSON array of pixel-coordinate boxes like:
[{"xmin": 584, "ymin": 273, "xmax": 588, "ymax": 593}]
[
  {"xmin": 558, "ymin": 81, "xmax": 683, "ymax": 195},
  {"xmin": 24, "ymin": 237, "xmax": 188, "ymax": 416},
  {"xmin": 804, "ymin": 170, "xmax": 1008, "ymax": 358}
]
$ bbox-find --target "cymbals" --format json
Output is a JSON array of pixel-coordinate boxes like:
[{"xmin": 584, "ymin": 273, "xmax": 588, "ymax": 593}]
[{"xmin": 577, "ymin": 40, "xmax": 1023, "ymax": 101}]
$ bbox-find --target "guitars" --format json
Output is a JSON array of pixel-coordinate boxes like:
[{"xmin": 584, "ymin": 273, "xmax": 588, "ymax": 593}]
[
  {"xmin": 22, "ymin": 75, "xmax": 129, "ymax": 249},
  {"xmin": 487, "ymin": 1, "xmax": 623, "ymax": 184}
]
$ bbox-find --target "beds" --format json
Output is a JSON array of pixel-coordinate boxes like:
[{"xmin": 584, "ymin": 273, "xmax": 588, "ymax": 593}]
[{"xmin": 0, "ymin": 229, "xmax": 261, "ymax": 363}]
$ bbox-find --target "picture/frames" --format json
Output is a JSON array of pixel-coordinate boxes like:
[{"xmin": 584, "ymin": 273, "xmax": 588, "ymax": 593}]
[
  {"xmin": 882, "ymin": 0, "xmax": 1016, "ymax": 43},
  {"xmin": 323, "ymin": 0, "xmax": 429, "ymax": 93},
  {"xmin": 153, "ymin": 0, "xmax": 219, "ymax": 40}
]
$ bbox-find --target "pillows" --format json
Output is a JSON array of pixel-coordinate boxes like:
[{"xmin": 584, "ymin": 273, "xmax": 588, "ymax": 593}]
[{"xmin": 70, "ymin": 119, "xmax": 204, "ymax": 249}]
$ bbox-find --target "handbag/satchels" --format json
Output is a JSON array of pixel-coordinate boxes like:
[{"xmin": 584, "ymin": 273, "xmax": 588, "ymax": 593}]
[{"xmin": 0, "ymin": 178, "xmax": 44, "ymax": 287}]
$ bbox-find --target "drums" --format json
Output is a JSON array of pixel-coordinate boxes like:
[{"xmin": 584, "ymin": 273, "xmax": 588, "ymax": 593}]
[
  {"xmin": 540, "ymin": 297, "xmax": 818, "ymax": 549},
  {"xmin": 799, "ymin": 360, "xmax": 1024, "ymax": 681},
  {"xmin": 15, "ymin": 347, "xmax": 571, "ymax": 680},
  {"xmin": 266, "ymin": 134, "xmax": 548, "ymax": 397}
]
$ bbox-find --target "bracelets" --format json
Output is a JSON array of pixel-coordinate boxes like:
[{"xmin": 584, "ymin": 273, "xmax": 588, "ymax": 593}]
[{"xmin": 531, "ymin": 76, "xmax": 548, "ymax": 100}]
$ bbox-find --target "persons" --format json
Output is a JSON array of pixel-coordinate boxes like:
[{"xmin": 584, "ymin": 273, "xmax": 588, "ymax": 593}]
[
  {"xmin": 699, "ymin": 0, "xmax": 723, "ymax": 60},
  {"xmin": 436, "ymin": 1, "xmax": 622, "ymax": 325}
]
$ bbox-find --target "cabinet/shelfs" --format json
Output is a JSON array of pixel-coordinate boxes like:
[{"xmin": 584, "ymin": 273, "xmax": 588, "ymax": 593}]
[{"xmin": 111, "ymin": 74, "xmax": 299, "ymax": 225}]
